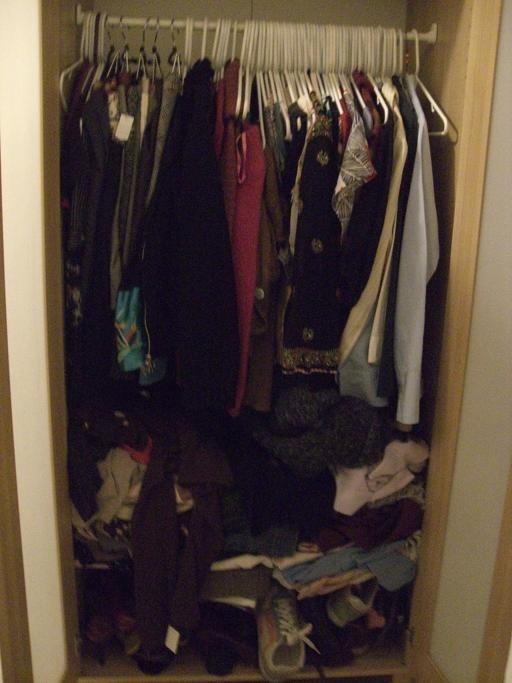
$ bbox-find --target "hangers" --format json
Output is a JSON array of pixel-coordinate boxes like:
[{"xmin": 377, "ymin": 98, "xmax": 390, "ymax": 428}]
[{"xmin": 59, "ymin": 6, "xmax": 449, "ymax": 142}]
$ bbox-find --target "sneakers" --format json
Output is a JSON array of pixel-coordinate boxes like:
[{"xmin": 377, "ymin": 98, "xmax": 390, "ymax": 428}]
[
  {"xmin": 326, "ymin": 579, "xmax": 379, "ymax": 628},
  {"xmin": 311, "ymin": 598, "xmax": 405, "ymax": 667},
  {"xmin": 256, "ymin": 578, "xmax": 321, "ymax": 683}
]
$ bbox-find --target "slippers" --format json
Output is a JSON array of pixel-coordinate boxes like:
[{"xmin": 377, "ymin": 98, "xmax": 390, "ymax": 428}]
[{"xmin": 123, "ymin": 609, "xmax": 255, "ymax": 675}]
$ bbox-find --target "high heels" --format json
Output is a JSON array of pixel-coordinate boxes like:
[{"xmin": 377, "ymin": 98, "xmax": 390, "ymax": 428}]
[
  {"xmin": 86, "ymin": 614, "xmax": 114, "ymax": 667},
  {"xmin": 112, "ymin": 606, "xmax": 138, "ymax": 654}
]
